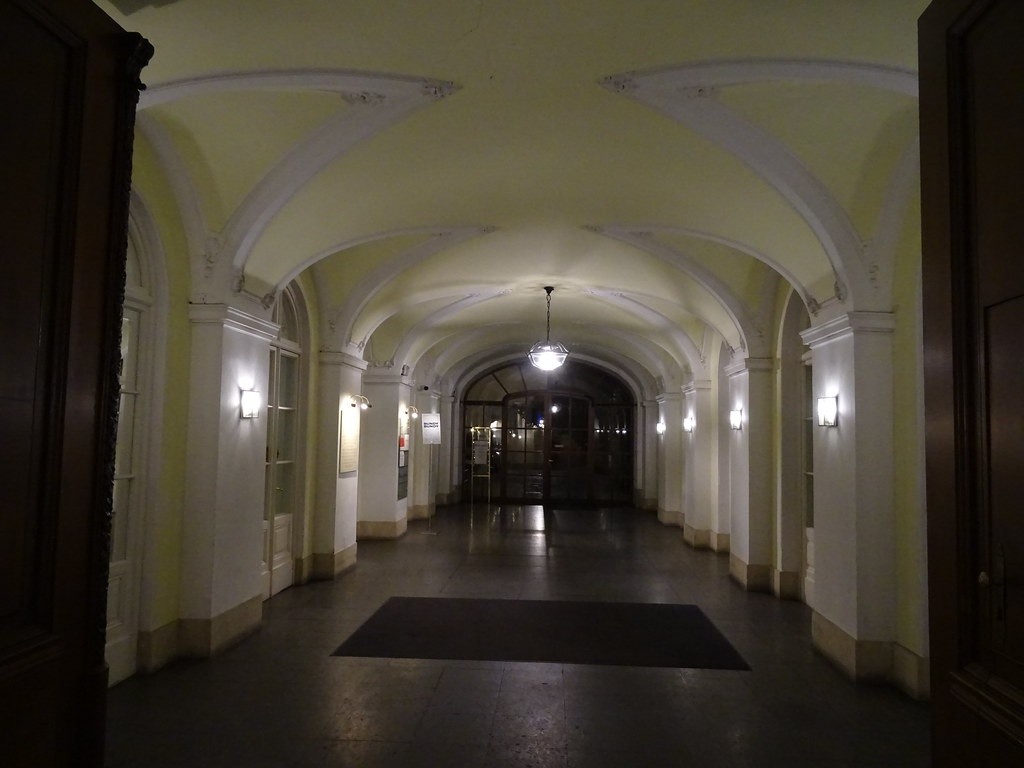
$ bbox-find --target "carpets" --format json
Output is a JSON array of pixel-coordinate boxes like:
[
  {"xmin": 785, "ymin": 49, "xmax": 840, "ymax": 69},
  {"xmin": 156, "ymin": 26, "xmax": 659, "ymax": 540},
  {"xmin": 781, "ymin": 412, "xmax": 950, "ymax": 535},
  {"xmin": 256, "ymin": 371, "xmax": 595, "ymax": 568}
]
[{"xmin": 329, "ymin": 596, "xmax": 751, "ymax": 671}]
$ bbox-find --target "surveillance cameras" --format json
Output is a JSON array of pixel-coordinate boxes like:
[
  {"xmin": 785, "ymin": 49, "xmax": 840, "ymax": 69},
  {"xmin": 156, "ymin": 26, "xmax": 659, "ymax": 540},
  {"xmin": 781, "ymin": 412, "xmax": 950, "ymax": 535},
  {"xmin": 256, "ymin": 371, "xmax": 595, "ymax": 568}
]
[{"xmin": 420, "ymin": 385, "xmax": 428, "ymax": 391}]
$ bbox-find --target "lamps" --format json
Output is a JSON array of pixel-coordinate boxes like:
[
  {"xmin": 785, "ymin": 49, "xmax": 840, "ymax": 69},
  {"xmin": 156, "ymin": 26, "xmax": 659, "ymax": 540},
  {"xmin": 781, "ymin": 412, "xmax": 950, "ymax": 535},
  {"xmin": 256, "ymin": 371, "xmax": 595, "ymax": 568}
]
[
  {"xmin": 728, "ymin": 410, "xmax": 741, "ymax": 431},
  {"xmin": 526, "ymin": 286, "xmax": 570, "ymax": 372},
  {"xmin": 351, "ymin": 394, "xmax": 372, "ymax": 412},
  {"xmin": 682, "ymin": 417, "xmax": 697, "ymax": 432},
  {"xmin": 408, "ymin": 406, "xmax": 421, "ymax": 422},
  {"xmin": 468, "ymin": 394, "xmax": 631, "ymax": 442},
  {"xmin": 239, "ymin": 385, "xmax": 262, "ymax": 419},
  {"xmin": 817, "ymin": 395, "xmax": 838, "ymax": 427}
]
